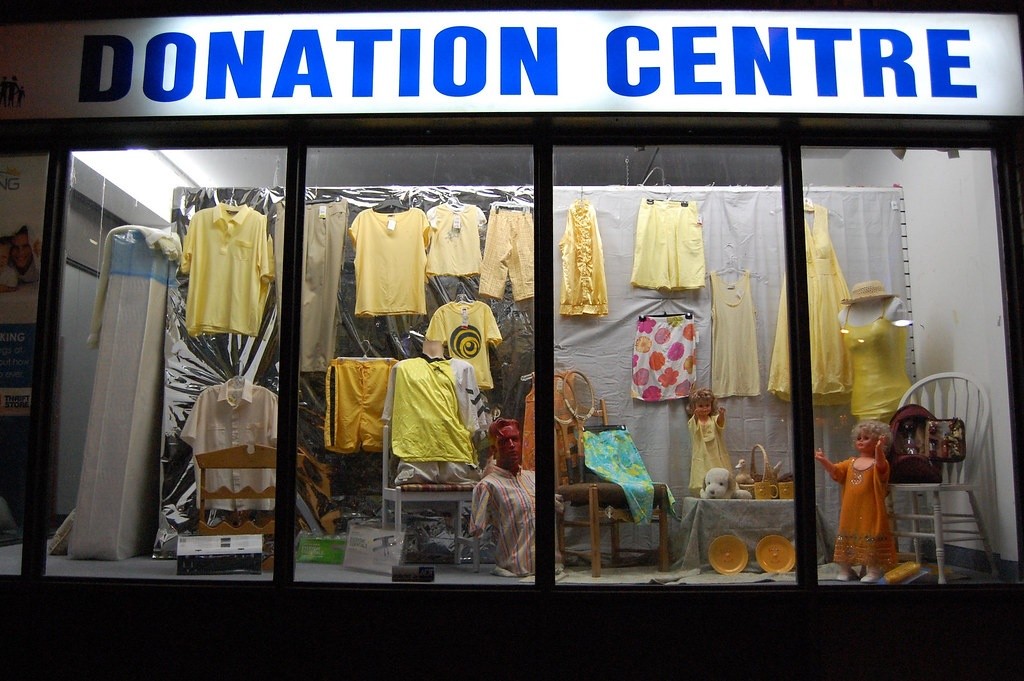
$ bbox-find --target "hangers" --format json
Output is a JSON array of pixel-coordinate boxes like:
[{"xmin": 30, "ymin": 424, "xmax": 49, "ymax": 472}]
[
  {"xmin": 453, "ymin": 281, "xmax": 473, "ymax": 304},
  {"xmin": 224, "ymin": 186, "xmax": 243, "ymax": 212},
  {"xmin": 705, "ymin": 244, "xmax": 760, "ymax": 279},
  {"xmin": 337, "ymin": 339, "xmax": 393, "ymax": 362},
  {"xmin": 519, "ymin": 356, "xmax": 586, "ymax": 383},
  {"xmin": 306, "ymin": 187, "xmax": 531, "ymax": 213},
  {"xmin": 230, "ymin": 360, "xmax": 245, "ymax": 396},
  {"xmin": 773, "ymin": 182, "xmax": 842, "ymax": 219},
  {"xmin": 638, "ymin": 297, "xmax": 693, "ymax": 321},
  {"xmin": 646, "ymin": 184, "xmax": 690, "ymax": 208}
]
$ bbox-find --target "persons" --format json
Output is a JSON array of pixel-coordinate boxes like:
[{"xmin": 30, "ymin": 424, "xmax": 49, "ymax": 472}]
[
  {"xmin": 838, "ymin": 279, "xmax": 914, "ymax": 422},
  {"xmin": 688, "ymin": 387, "xmax": 732, "ymax": 494},
  {"xmin": 815, "ymin": 420, "xmax": 898, "ymax": 583},
  {"xmin": 0, "ymin": 227, "xmax": 38, "ymax": 291},
  {"xmin": 381, "ymin": 340, "xmax": 484, "ymax": 485},
  {"xmin": 469, "ymin": 420, "xmax": 562, "ymax": 575}
]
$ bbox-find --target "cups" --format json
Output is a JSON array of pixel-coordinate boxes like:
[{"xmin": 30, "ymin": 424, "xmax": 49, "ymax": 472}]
[
  {"xmin": 779, "ymin": 481, "xmax": 793, "ymax": 499},
  {"xmin": 754, "ymin": 482, "xmax": 778, "ymax": 499}
]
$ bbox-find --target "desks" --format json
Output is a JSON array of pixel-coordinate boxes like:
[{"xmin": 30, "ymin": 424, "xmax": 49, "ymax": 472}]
[{"xmin": 677, "ymin": 496, "xmax": 828, "ymax": 571}]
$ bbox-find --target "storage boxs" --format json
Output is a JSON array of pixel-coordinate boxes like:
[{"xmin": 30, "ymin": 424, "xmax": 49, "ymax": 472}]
[{"xmin": 176, "ymin": 534, "xmax": 264, "ymax": 577}]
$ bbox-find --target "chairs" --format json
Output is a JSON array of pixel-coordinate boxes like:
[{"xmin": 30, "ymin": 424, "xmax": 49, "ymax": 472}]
[
  {"xmin": 887, "ymin": 370, "xmax": 1001, "ymax": 584},
  {"xmin": 553, "ymin": 398, "xmax": 668, "ymax": 579},
  {"xmin": 381, "ymin": 426, "xmax": 482, "ymax": 572}
]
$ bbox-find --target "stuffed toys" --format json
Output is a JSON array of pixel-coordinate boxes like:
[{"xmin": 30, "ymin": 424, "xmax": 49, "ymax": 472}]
[{"xmin": 700, "ymin": 468, "xmax": 751, "ymax": 499}]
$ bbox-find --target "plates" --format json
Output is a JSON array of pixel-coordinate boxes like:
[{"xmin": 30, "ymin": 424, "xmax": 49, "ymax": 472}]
[
  {"xmin": 708, "ymin": 535, "xmax": 748, "ymax": 576},
  {"xmin": 755, "ymin": 535, "xmax": 795, "ymax": 574}
]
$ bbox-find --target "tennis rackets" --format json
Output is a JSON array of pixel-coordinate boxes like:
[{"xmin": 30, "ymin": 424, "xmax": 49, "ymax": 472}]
[
  {"xmin": 551, "ymin": 374, "xmax": 578, "ymax": 484},
  {"xmin": 561, "ymin": 369, "xmax": 596, "ymax": 484}
]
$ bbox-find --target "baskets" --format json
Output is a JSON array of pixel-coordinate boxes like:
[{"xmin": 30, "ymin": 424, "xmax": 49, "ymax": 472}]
[{"xmin": 738, "ymin": 444, "xmax": 778, "ymax": 499}]
